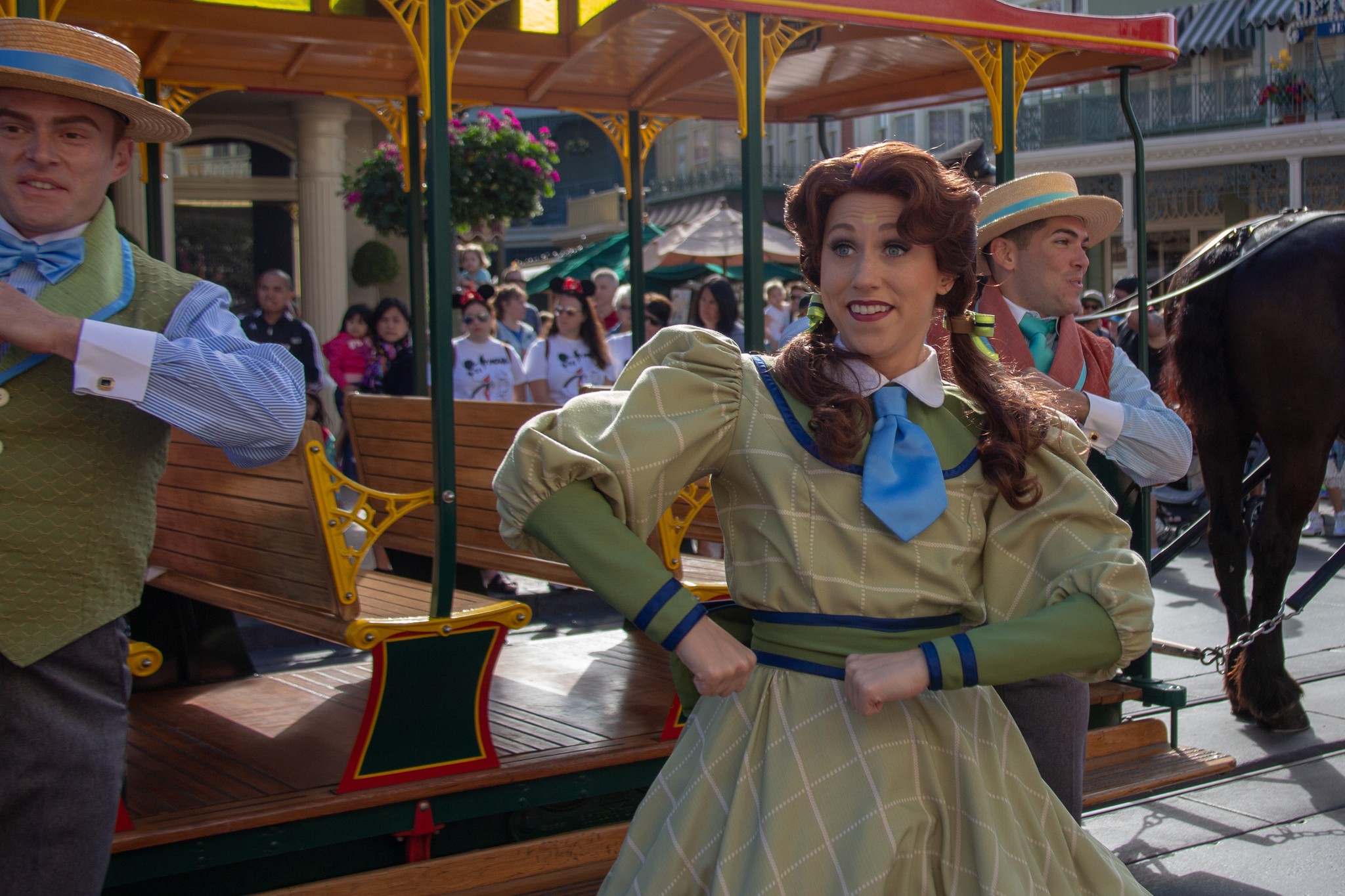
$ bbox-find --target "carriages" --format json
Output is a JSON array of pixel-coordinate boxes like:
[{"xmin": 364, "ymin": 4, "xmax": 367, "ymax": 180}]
[{"xmin": 0, "ymin": 0, "xmax": 1344, "ymax": 894}]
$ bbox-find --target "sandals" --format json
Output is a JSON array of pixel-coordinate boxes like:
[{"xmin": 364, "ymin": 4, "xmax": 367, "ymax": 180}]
[{"xmin": 487, "ymin": 573, "xmax": 516, "ymax": 593}]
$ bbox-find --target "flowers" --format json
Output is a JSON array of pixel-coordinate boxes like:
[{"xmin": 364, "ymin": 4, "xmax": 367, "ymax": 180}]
[
  {"xmin": 328, "ymin": 108, "xmax": 562, "ymax": 253},
  {"xmin": 1257, "ymin": 48, "xmax": 1321, "ymax": 114}
]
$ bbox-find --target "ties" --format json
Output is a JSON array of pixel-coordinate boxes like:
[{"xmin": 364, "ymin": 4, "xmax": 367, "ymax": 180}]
[
  {"xmin": 1017, "ymin": 317, "xmax": 1056, "ymax": 376},
  {"xmin": 862, "ymin": 386, "xmax": 947, "ymax": 540}
]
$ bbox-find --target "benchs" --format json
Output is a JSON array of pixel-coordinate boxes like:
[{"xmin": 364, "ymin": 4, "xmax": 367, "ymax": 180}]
[
  {"xmin": 341, "ymin": 389, "xmax": 751, "ymax": 743},
  {"xmin": 576, "ymin": 381, "xmax": 724, "ymax": 545},
  {"xmin": 141, "ymin": 420, "xmax": 533, "ymax": 794}
]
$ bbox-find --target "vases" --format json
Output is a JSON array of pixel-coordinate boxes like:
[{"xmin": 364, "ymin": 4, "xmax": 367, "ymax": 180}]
[{"xmin": 1284, "ymin": 112, "xmax": 1305, "ymax": 125}]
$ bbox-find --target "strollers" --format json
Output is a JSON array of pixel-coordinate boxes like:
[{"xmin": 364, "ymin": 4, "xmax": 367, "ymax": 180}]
[{"xmin": 1152, "ymin": 481, "xmax": 1266, "ymax": 547}]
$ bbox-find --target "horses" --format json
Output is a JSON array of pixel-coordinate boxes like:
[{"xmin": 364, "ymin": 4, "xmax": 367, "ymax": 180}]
[{"xmin": 1164, "ymin": 209, "xmax": 1345, "ymax": 733}]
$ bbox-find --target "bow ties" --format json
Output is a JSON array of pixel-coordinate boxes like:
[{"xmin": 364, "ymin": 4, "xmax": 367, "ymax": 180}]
[{"xmin": 0, "ymin": 228, "xmax": 86, "ymax": 285}]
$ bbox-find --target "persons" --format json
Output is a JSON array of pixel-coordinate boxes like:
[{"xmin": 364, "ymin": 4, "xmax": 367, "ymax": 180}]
[
  {"xmin": 304, "ymin": 389, "xmax": 346, "ymax": 511},
  {"xmin": 360, "ymin": 295, "xmax": 415, "ymax": 396},
  {"xmin": 534, "ymin": 308, "xmax": 553, "ymax": 341},
  {"xmin": 457, "ymin": 243, "xmax": 492, "ymax": 291},
  {"xmin": 517, "ymin": 278, "xmax": 617, "ymax": 408},
  {"xmin": 234, "ymin": 268, "xmax": 326, "ymax": 392},
  {"xmin": 488, "ymin": 284, "xmax": 537, "ymax": 367},
  {"xmin": 492, "ymin": 266, "xmax": 542, "ymax": 340},
  {"xmin": 425, "ymin": 284, "xmax": 527, "ymax": 403},
  {"xmin": 602, "ymin": 260, "xmax": 820, "ymax": 379},
  {"xmin": 1079, "ymin": 276, "xmax": 1344, "ymax": 540},
  {"xmin": 1, "ymin": 17, "xmax": 309, "ymax": 894},
  {"xmin": 587, "ymin": 266, "xmax": 624, "ymax": 340},
  {"xmin": 320, "ymin": 302, "xmax": 377, "ymax": 471},
  {"xmin": 491, "ymin": 137, "xmax": 1157, "ymax": 893},
  {"xmin": 966, "ymin": 168, "xmax": 1195, "ymax": 835}
]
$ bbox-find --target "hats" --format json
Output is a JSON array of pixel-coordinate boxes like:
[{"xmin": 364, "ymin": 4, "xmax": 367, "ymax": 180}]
[
  {"xmin": 975, "ymin": 171, "xmax": 1124, "ymax": 276},
  {"xmin": 1, "ymin": 17, "xmax": 193, "ymax": 144},
  {"xmin": 938, "ymin": 138, "xmax": 997, "ymax": 179},
  {"xmin": 1080, "ymin": 289, "xmax": 1105, "ymax": 309}
]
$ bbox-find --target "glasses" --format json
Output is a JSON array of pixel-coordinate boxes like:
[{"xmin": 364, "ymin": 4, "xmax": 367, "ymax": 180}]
[
  {"xmin": 552, "ymin": 304, "xmax": 582, "ymax": 317},
  {"xmin": 462, "ymin": 313, "xmax": 490, "ymax": 323},
  {"xmin": 1082, "ymin": 304, "xmax": 1100, "ymax": 310}
]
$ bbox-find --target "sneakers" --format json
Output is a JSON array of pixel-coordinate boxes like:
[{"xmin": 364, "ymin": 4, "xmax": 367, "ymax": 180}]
[{"xmin": 1301, "ymin": 511, "xmax": 1345, "ymax": 537}]
